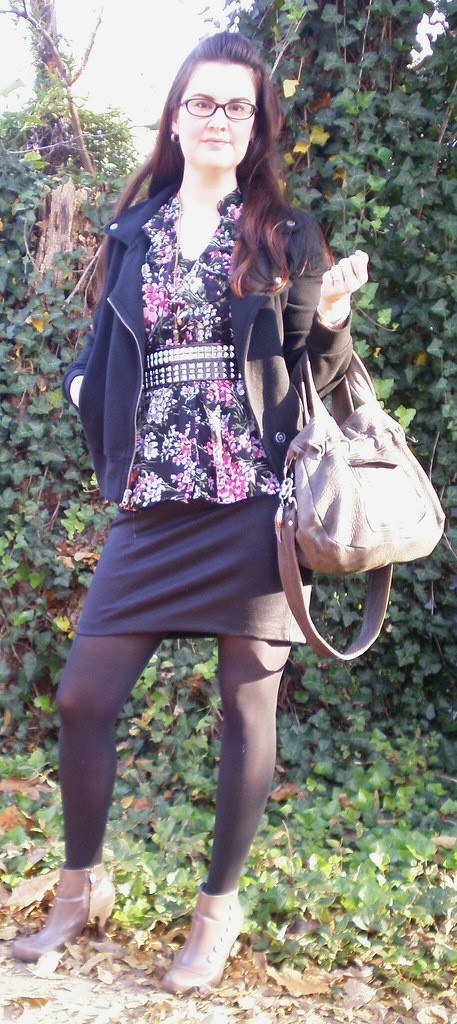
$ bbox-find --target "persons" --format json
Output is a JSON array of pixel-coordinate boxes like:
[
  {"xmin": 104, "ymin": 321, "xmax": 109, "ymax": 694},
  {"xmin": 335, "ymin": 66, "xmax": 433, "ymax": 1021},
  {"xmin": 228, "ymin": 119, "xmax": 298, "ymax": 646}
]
[{"xmin": 15, "ymin": 34, "xmax": 370, "ymax": 993}]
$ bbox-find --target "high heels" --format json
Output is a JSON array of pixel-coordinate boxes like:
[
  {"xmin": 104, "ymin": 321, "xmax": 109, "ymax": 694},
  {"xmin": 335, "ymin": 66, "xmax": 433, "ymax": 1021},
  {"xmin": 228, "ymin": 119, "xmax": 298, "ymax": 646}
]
[
  {"xmin": 14, "ymin": 863, "xmax": 115, "ymax": 962},
  {"xmin": 162, "ymin": 881, "xmax": 244, "ymax": 993}
]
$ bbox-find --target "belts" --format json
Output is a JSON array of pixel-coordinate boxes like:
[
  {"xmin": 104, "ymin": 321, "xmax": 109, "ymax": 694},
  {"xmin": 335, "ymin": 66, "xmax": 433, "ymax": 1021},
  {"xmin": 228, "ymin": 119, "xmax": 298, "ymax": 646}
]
[{"xmin": 142, "ymin": 344, "xmax": 242, "ymax": 390}]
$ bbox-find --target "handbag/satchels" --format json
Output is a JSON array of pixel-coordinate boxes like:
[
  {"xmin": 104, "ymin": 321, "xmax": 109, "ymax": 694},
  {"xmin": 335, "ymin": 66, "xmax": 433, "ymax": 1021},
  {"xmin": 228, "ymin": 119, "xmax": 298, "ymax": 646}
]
[{"xmin": 276, "ymin": 349, "xmax": 446, "ymax": 660}]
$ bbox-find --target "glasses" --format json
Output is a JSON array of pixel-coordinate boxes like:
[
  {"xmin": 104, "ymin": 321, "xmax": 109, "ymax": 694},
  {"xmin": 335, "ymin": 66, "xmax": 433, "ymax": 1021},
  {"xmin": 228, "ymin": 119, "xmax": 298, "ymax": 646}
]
[{"xmin": 176, "ymin": 99, "xmax": 259, "ymax": 120}]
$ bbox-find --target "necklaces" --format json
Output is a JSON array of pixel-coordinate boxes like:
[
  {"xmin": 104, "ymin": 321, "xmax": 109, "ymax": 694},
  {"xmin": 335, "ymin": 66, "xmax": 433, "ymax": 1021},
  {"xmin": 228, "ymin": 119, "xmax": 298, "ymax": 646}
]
[{"xmin": 173, "ymin": 190, "xmax": 182, "ymax": 345}]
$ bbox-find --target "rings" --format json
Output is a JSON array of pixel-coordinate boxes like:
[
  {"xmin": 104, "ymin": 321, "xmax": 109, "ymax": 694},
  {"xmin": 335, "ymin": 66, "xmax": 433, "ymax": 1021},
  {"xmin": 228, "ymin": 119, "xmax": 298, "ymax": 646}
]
[
  {"xmin": 334, "ymin": 276, "xmax": 343, "ymax": 282},
  {"xmin": 345, "ymin": 272, "xmax": 354, "ymax": 277}
]
